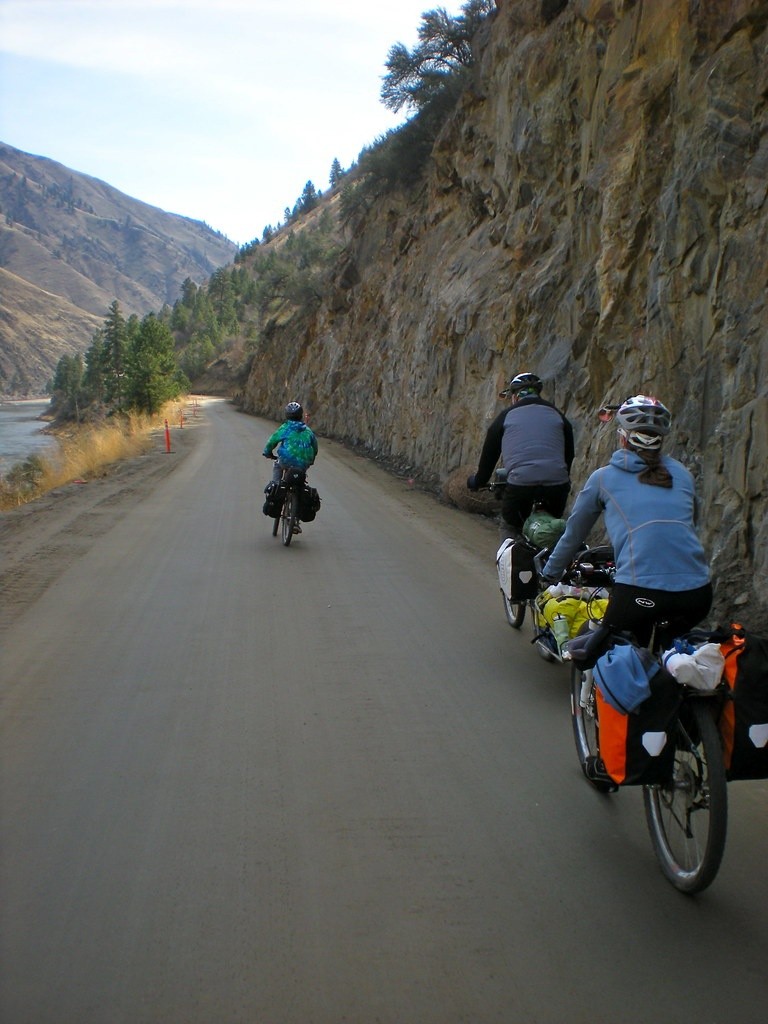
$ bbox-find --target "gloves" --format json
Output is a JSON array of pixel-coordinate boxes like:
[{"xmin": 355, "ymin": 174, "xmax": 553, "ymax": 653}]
[
  {"xmin": 266, "ymin": 453, "xmax": 273, "ymax": 459},
  {"xmin": 466, "ymin": 475, "xmax": 481, "ymax": 493},
  {"xmin": 544, "ymin": 575, "xmax": 560, "ymax": 586}
]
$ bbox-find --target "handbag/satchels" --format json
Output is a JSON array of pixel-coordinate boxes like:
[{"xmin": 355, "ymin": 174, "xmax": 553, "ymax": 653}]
[
  {"xmin": 722, "ymin": 621, "xmax": 768, "ymax": 781},
  {"xmin": 295, "ymin": 485, "xmax": 321, "ymax": 522},
  {"xmin": 496, "ymin": 536, "xmax": 537, "ymax": 601},
  {"xmin": 595, "ymin": 660, "xmax": 673, "ymax": 787},
  {"xmin": 263, "ymin": 481, "xmax": 287, "ymax": 518}
]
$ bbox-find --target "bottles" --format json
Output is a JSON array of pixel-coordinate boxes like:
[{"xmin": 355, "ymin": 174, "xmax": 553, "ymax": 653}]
[{"xmin": 551, "ymin": 611, "xmax": 570, "ymax": 662}]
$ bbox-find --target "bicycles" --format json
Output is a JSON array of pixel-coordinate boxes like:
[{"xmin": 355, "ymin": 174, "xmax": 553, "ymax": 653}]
[
  {"xmin": 477, "ymin": 467, "xmax": 590, "ymax": 662},
  {"xmin": 262, "ymin": 451, "xmax": 315, "ymax": 547},
  {"xmin": 538, "ymin": 544, "xmax": 738, "ymax": 894}
]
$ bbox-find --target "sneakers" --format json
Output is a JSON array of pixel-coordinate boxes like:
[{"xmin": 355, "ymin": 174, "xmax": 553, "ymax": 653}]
[{"xmin": 293, "ymin": 524, "xmax": 302, "ymax": 533}]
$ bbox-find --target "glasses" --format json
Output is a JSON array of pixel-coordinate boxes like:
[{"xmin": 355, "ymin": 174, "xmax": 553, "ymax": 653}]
[{"xmin": 598, "ymin": 410, "xmax": 613, "ymax": 416}]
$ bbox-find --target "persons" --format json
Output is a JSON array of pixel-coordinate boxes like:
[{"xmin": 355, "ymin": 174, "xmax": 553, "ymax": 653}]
[
  {"xmin": 263, "ymin": 402, "xmax": 319, "ymax": 533},
  {"xmin": 474, "ymin": 373, "xmax": 574, "ymax": 545},
  {"xmin": 542, "ymin": 394, "xmax": 713, "ymax": 788}
]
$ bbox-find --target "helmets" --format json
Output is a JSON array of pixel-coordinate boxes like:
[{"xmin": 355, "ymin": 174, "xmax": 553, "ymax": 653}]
[
  {"xmin": 509, "ymin": 372, "xmax": 543, "ymax": 393},
  {"xmin": 617, "ymin": 394, "xmax": 672, "ymax": 435},
  {"xmin": 285, "ymin": 402, "xmax": 303, "ymax": 421}
]
